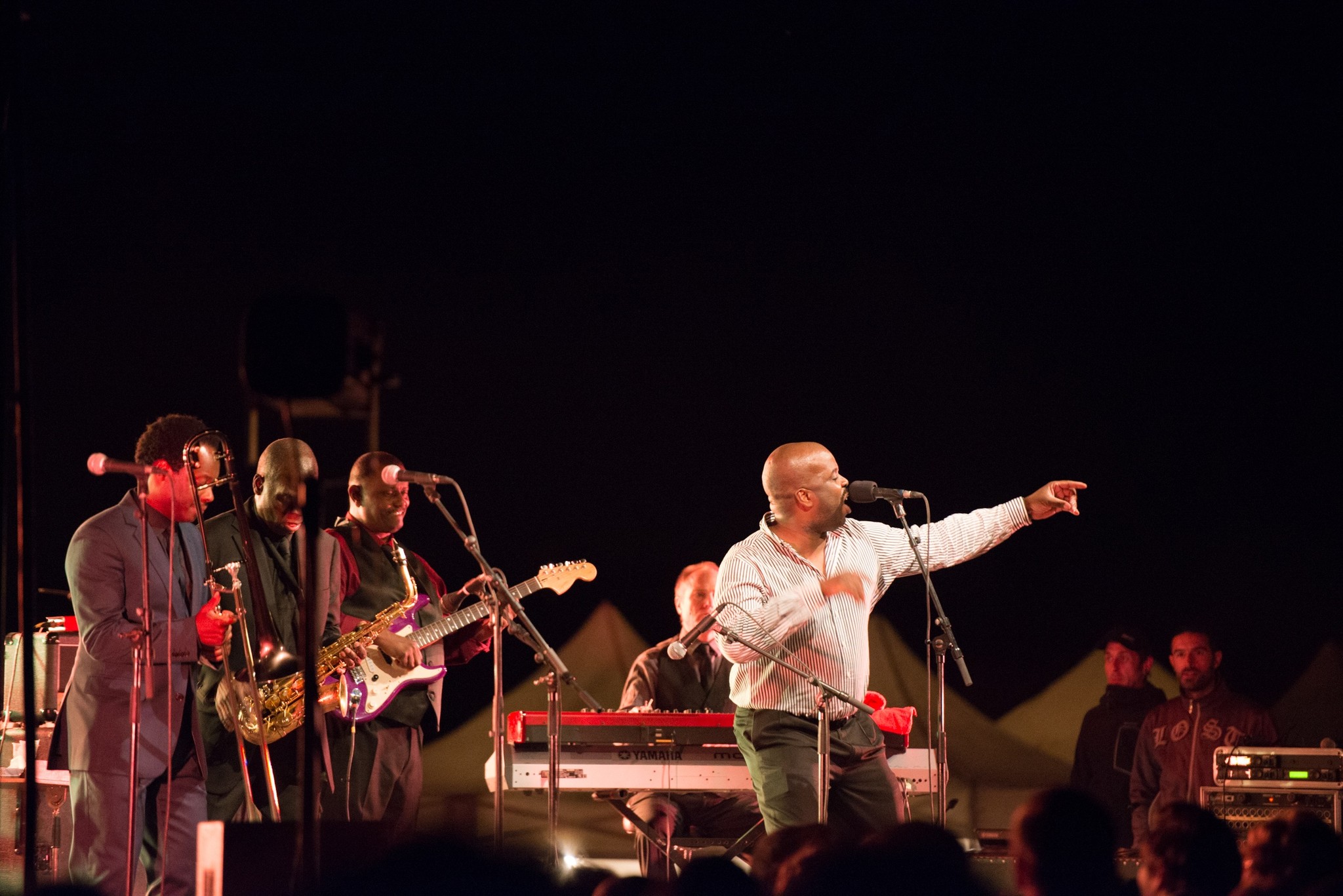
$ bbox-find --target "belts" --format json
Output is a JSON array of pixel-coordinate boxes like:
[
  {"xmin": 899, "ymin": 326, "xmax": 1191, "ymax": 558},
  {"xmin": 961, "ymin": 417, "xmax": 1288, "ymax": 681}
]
[{"xmin": 807, "ymin": 715, "xmax": 854, "ymax": 731}]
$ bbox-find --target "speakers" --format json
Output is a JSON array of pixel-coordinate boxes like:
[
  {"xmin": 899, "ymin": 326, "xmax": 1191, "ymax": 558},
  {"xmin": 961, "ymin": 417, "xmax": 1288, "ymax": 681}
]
[{"xmin": 1199, "ymin": 786, "xmax": 1343, "ymax": 841}]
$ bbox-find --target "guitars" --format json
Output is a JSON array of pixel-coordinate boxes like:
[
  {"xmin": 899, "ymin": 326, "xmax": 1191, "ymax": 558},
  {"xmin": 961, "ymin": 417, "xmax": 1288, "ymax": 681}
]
[{"xmin": 322, "ymin": 558, "xmax": 600, "ymax": 725}]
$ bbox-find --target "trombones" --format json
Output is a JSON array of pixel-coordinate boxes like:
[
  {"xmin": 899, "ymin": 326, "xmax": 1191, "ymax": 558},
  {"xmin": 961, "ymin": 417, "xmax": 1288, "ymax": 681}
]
[{"xmin": 182, "ymin": 428, "xmax": 282, "ymax": 827}]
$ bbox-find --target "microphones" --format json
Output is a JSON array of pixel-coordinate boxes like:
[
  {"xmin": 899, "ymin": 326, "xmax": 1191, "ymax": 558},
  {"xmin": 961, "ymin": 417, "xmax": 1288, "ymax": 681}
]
[
  {"xmin": 381, "ymin": 465, "xmax": 454, "ymax": 485},
  {"xmin": 849, "ymin": 480, "xmax": 923, "ymax": 503},
  {"xmin": 87, "ymin": 453, "xmax": 169, "ymax": 477},
  {"xmin": 667, "ymin": 603, "xmax": 726, "ymax": 659}
]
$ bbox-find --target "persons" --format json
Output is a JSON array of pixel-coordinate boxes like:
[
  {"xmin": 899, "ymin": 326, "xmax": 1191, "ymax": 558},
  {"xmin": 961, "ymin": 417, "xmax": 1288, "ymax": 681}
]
[
  {"xmin": 319, "ymin": 452, "xmax": 519, "ymax": 832},
  {"xmin": 620, "ymin": 562, "xmax": 760, "ymax": 882},
  {"xmin": 1071, "ymin": 630, "xmax": 1167, "ymax": 849},
  {"xmin": 1131, "ymin": 625, "xmax": 1277, "ymax": 849},
  {"xmin": 709, "ymin": 441, "xmax": 1087, "ymax": 837},
  {"xmin": 48, "ymin": 416, "xmax": 238, "ymax": 896},
  {"xmin": 220, "ymin": 798, "xmax": 1343, "ymax": 896},
  {"xmin": 198, "ymin": 438, "xmax": 319, "ymax": 821}
]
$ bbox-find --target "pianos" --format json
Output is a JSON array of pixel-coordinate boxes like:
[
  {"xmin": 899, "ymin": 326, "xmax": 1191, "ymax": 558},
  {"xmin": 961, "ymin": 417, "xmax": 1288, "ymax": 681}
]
[{"xmin": 483, "ymin": 698, "xmax": 950, "ymax": 876}]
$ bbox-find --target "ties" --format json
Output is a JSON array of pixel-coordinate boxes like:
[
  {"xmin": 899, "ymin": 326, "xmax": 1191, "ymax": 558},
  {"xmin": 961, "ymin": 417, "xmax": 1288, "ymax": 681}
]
[
  {"xmin": 272, "ymin": 535, "xmax": 294, "ymax": 632},
  {"xmin": 379, "ymin": 547, "xmax": 400, "ymax": 573},
  {"xmin": 165, "ymin": 524, "xmax": 187, "ymax": 601},
  {"xmin": 700, "ymin": 644, "xmax": 715, "ymax": 694}
]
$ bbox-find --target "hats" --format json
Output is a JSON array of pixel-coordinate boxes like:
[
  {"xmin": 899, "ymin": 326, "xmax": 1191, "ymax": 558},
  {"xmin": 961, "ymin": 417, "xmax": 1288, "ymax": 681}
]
[{"xmin": 1105, "ymin": 621, "xmax": 1156, "ymax": 655}]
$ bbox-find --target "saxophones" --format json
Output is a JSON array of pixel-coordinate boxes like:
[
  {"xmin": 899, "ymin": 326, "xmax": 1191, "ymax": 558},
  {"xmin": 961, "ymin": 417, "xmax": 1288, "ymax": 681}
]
[{"xmin": 232, "ymin": 535, "xmax": 419, "ymax": 749}]
